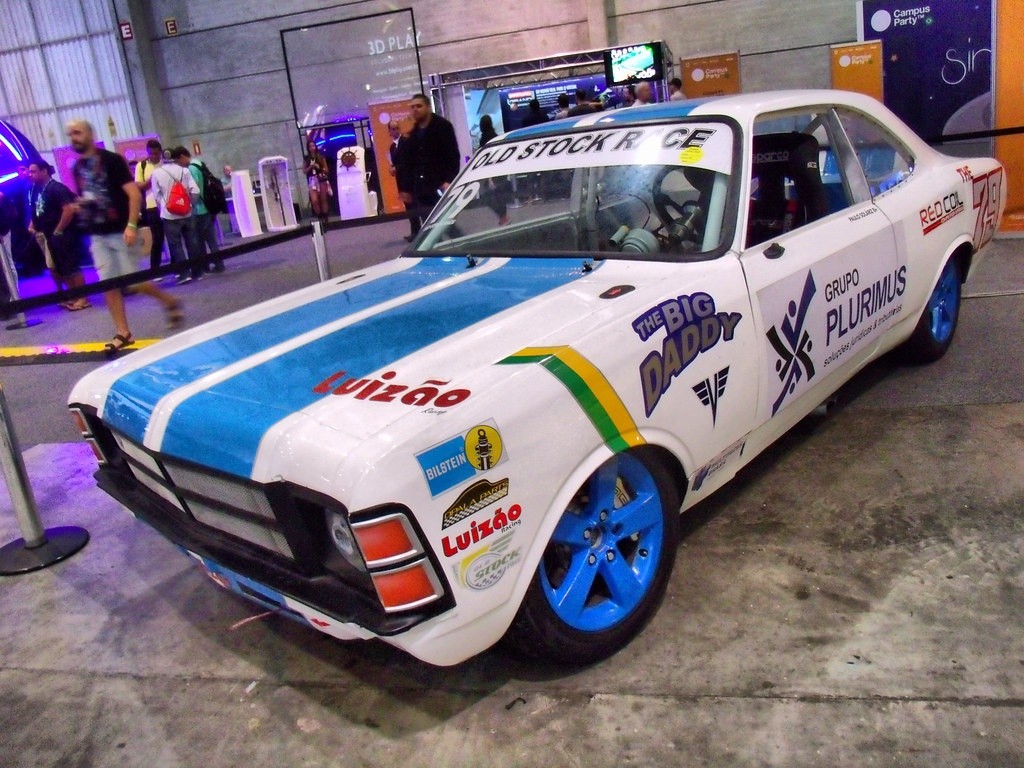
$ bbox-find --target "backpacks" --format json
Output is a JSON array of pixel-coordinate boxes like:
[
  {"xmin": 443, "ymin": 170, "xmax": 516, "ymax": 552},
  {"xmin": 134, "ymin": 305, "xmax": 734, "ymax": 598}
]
[
  {"xmin": 187, "ymin": 160, "xmax": 229, "ymax": 216},
  {"xmin": 157, "ymin": 167, "xmax": 193, "ymax": 217}
]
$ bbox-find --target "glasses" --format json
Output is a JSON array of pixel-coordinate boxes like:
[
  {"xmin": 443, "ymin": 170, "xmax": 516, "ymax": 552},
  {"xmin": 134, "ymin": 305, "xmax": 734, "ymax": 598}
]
[{"xmin": 410, "ymin": 104, "xmax": 426, "ymax": 108}]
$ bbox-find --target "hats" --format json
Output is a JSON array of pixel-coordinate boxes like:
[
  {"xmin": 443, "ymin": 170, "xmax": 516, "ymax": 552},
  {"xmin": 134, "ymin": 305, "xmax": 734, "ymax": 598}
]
[
  {"xmin": 162, "ymin": 147, "xmax": 176, "ymax": 162},
  {"xmin": 668, "ymin": 78, "xmax": 682, "ymax": 87}
]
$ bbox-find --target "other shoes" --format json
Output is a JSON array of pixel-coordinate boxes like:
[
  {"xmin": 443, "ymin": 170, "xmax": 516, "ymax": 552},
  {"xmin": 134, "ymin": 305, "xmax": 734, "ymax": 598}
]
[
  {"xmin": 497, "ymin": 214, "xmax": 512, "ymax": 226},
  {"xmin": 149, "ymin": 277, "xmax": 163, "ymax": 282},
  {"xmin": 204, "ymin": 267, "xmax": 223, "ymax": 275},
  {"xmin": 174, "ymin": 273, "xmax": 203, "ymax": 285},
  {"xmin": 403, "ymin": 232, "xmax": 417, "ymax": 241}
]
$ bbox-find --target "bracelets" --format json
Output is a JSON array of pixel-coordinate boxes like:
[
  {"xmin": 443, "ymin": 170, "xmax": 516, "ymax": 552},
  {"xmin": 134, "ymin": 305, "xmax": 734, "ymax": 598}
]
[{"xmin": 127, "ymin": 222, "xmax": 137, "ymax": 230}]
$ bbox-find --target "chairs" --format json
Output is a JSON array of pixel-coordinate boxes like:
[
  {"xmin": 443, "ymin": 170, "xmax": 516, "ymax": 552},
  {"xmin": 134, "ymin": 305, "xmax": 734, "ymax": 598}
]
[{"xmin": 744, "ymin": 132, "xmax": 827, "ymax": 249}]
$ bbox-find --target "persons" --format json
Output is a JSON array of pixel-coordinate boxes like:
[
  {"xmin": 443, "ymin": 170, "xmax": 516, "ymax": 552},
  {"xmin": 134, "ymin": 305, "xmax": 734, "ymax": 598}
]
[
  {"xmin": 669, "ymin": 77, "xmax": 687, "ymax": 100},
  {"xmin": 555, "ymin": 93, "xmax": 570, "ymax": 119},
  {"xmin": 567, "ymin": 89, "xmax": 596, "ymax": 117},
  {"xmin": 522, "ymin": 100, "xmax": 549, "ymax": 127},
  {"xmin": 623, "ymin": 81, "xmax": 651, "ymax": 107},
  {"xmin": 220, "ymin": 166, "xmax": 239, "ymax": 233},
  {"xmin": 306, "ymin": 140, "xmax": 334, "ymax": 234},
  {"xmin": 387, "ymin": 94, "xmax": 461, "ymax": 242},
  {"xmin": 478, "ymin": 115, "xmax": 512, "ymax": 226},
  {"xmin": 17, "ymin": 119, "xmax": 226, "ymax": 354}
]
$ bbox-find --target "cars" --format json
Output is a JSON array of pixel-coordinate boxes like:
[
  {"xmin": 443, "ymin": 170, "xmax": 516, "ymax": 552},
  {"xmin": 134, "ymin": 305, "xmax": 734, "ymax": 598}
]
[{"xmin": 65, "ymin": 89, "xmax": 1007, "ymax": 670}]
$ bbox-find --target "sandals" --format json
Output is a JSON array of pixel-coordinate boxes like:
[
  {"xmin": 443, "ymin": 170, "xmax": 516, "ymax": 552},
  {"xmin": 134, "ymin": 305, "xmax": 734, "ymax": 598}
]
[
  {"xmin": 161, "ymin": 302, "xmax": 187, "ymax": 330},
  {"xmin": 102, "ymin": 332, "xmax": 136, "ymax": 352}
]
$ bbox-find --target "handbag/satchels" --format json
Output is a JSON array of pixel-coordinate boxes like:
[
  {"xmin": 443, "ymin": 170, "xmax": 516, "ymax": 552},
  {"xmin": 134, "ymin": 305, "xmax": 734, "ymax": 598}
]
[{"xmin": 34, "ymin": 231, "xmax": 56, "ymax": 269}]
